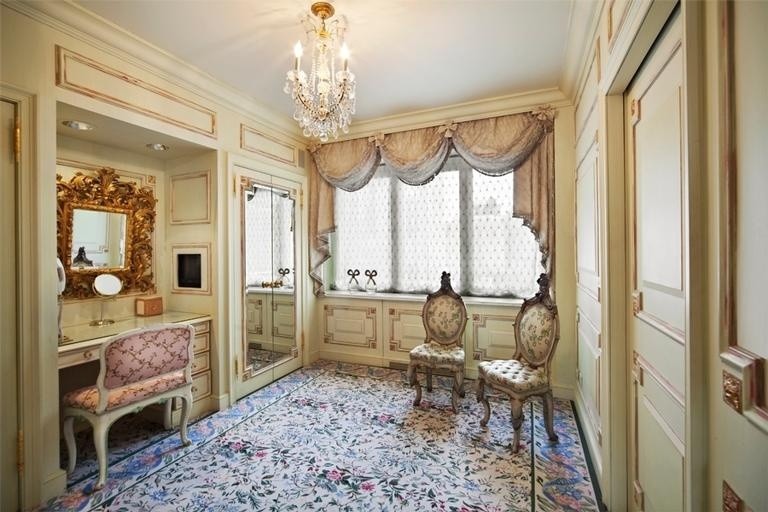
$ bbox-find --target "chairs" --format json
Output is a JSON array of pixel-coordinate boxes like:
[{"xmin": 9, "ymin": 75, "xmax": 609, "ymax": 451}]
[
  {"xmin": 476, "ymin": 272, "xmax": 561, "ymax": 454},
  {"xmin": 408, "ymin": 271, "xmax": 468, "ymax": 415}
]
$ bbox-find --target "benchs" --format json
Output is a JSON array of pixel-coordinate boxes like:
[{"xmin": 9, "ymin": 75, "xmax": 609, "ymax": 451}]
[{"xmin": 61, "ymin": 321, "xmax": 196, "ymax": 492}]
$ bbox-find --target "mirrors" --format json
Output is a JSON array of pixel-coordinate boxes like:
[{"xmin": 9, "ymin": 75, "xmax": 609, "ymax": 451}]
[
  {"xmin": 56, "ymin": 166, "xmax": 159, "ymax": 300},
  {"xmin": 90, "ymin": 273, "xmax": 124, "ymax": 326},
  {"xmin": 240, "ymin": 175, "xmax": 298, "ymax": 382}
]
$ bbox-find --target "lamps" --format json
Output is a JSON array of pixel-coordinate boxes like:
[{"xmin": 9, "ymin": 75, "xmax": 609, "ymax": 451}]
[{"xmin": 283, "ymin": 0, "xmax": 357, "ymax": 142}]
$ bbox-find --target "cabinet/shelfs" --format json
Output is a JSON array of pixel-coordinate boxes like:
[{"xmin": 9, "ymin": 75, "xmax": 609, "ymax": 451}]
[
  {"xmin": 466, "ymin": 304, "xmax": 521, "ymax": 380},
  {"xmin": 164, "ymin": 310, "xmax": 213, "ymax": 432},
  {"xmin": 246, "ymin": 292, "xmax": 295, "ymax": 355},
  {"xmin": 383, "ymin": 301, "xmax": 466, "ymax": 379},
  {"xmin": 317, "ymin": 298, "xmax": 383, "ymax": 368}
]
